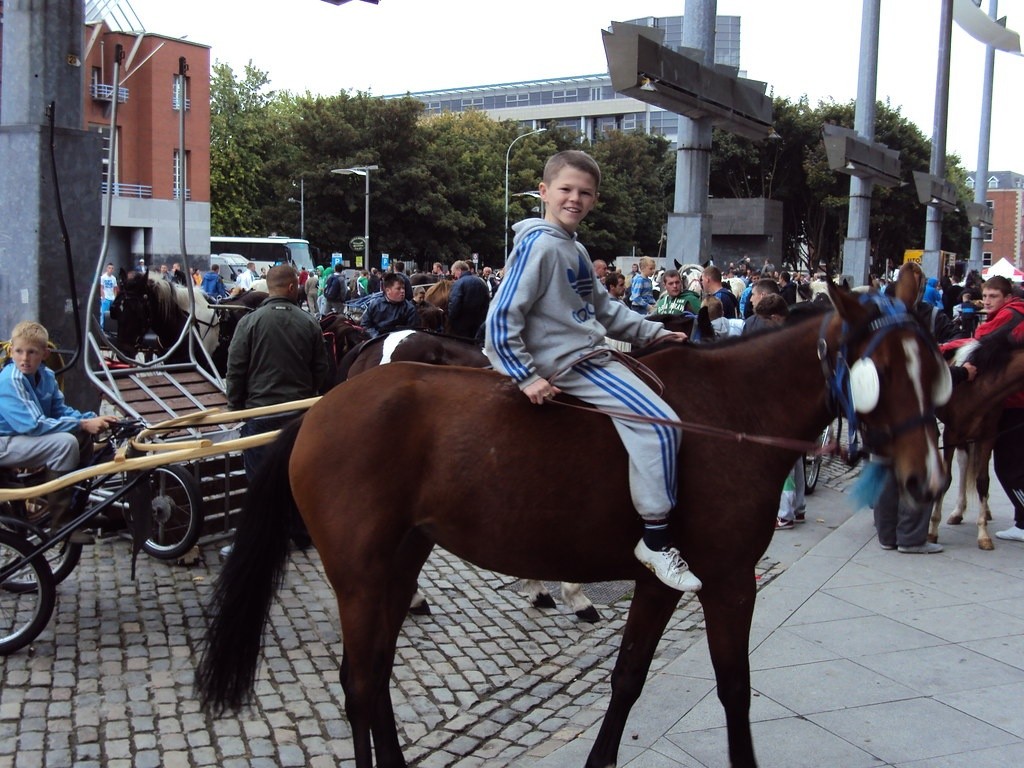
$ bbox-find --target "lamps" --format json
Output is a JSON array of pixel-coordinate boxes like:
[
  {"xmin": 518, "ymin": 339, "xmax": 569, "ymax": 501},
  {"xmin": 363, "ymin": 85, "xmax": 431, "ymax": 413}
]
[
  {"xmin": 845, "ymin": 162, "xmax": 855, "ymax": 169},
  {"xmin": 897, "ymin": 181, "xmax": 909, "ymax": 187},
  {"xmin": 930, "ymin": 198, "xmax": 939, "ymax": 204},
  {"xmin": 979, "ymin": 221, "xmax": 985, "ymax": 227},
  {"xmin": 768, "ymin": 126, "xmax": 782, "ymax": 138},
  {"xmin": 992, "ymin": 225, "xmax": 998, "ymax": 230},
  {"xmin": 638, "ymin": 73, "xmax": 657, "ymax": 93},
  {"xmin": 955, "ymin": 208, "xmax": 960, "ymax": 213}
]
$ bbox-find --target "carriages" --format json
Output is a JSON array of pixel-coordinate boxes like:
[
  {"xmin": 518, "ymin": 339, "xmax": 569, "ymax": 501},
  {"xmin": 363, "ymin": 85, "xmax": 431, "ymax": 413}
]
[{"xmin": 0, "ymin": 308, "xmax": 715, "ymax": 655}]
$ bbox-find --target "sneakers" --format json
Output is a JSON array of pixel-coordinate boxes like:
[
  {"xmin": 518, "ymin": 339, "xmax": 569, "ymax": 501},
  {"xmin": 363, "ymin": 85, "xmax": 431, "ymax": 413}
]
[
  {"xmin": 775, "ymin": 517, "xmax": 793, "ymax": 529},
  {"xmin": 995, "ymin": 525, "xmax": 1024, "ymax": 541},
  {"xmin": 794, "ymin": 512, "xmax": 804, "ymax": 523},
  {"xmin": 633, "ymin": 538, "xmax": 701, "ymax": 592}
]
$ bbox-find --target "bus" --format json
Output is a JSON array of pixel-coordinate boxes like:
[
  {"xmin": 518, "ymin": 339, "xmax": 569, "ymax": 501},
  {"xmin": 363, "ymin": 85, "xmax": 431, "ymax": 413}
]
[{"xmin": 210, "ymin": 235, "xmax": 315, "ymax": 276}]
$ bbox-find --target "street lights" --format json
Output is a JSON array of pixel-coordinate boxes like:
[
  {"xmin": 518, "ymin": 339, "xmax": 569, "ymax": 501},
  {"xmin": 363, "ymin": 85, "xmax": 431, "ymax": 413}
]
[
  {"xmin": 332, "ymin": 166, "xmax": 370, "ymax": 271},
  {"xmin": 288, "ymin": 199, "xmax": 304, "ymax": 239},
  {"xmin": 505, "ymin": 129, "xmax": 546, "ymax": 264},
  {"xmin": 512, "ymin": 192, "xmax": 544, "ymax": 219}
]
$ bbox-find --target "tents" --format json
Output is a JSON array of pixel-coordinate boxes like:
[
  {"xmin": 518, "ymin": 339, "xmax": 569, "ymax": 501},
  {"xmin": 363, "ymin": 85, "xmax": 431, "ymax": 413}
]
[{"xmin": 977, "ymin": 255, "xmax": 1024, "ymax": 282}]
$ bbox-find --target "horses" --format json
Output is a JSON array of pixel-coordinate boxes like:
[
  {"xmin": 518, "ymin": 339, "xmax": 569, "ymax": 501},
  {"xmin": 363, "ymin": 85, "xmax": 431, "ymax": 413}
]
[
  {"xmin": 927, "ymin": 337, "xmax": 1024, "ymax": 551},
  {"xmin": 191, "ymin": 264, "xmax": 950, "ymax": 768},
  {"xmin": 110, "ymin": 267, "xmax": 269, "ymax": 378},
  {"xmin": 328, "ymin": 302, "xmax": 717, "ymax": 623},
  {"xmin": 321, "ymin": 273, "xmax": 458, "ymax": 359}
]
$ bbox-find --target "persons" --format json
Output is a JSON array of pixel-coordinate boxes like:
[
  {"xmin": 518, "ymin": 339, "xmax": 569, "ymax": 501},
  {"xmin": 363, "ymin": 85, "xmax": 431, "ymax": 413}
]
[
  {"xmin": 81, "ymin": 246, "xmax": 1024, "ymax": 583},
  {"xmin": 200, "ymin": 263, "xmax": 229, "ymax": 302},
  {"xmin": 656, "ymin": 270, "xmax": 702, "ymax": 316},
  {"xmin": 0, "ymin": 320, "xmax": 123, "ymax": 544},
  {"xmin": 101, "ymin": 262, "xmax": 119, "ymax": 333},
  {"xmin": 445, "ymin": 260, "xmax": 489, "ymax": 343},
  {"xmin": 226, "ymin": 263, "xmax": 334, "ymax": 550},
  {"xmin": 359, "ymin": 275, "xmax": 418, "ymax": 340},
  {"xmin": 483, "ymin": 150, "xmax": 703, "ymax": 593},
  {"xmin": 629, "ymin": 256, "xmax": 657, "ymax": 315}
]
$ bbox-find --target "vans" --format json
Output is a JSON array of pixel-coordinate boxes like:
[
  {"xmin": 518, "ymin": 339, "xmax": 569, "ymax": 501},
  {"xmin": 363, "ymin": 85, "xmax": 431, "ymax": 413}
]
[{"xmin": 210, "ymin": 252, "xmax": 259, "ymax": 280}]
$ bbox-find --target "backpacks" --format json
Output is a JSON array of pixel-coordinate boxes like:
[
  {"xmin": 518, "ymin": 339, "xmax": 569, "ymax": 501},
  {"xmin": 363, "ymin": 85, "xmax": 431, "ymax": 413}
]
[{"xmin": 324, "ymin": 274, "xmax": 344, "ymax": 300}]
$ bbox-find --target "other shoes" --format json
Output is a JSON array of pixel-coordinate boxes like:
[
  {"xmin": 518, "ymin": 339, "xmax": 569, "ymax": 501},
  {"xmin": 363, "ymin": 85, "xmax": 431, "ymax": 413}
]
[
  {"xmin": 897, "ymin": 541, "xmax": 942, "ymax": 553},
  {"xmin": 881, "ymin": 544, "xmax": 896, "ymax": 549}
]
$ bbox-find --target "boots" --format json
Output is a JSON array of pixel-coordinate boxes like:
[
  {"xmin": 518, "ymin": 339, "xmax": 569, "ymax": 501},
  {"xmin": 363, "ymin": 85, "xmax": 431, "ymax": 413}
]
[{"xmin": 49, "ymin": 468, "xmax": 96, "ymax": 545}]
